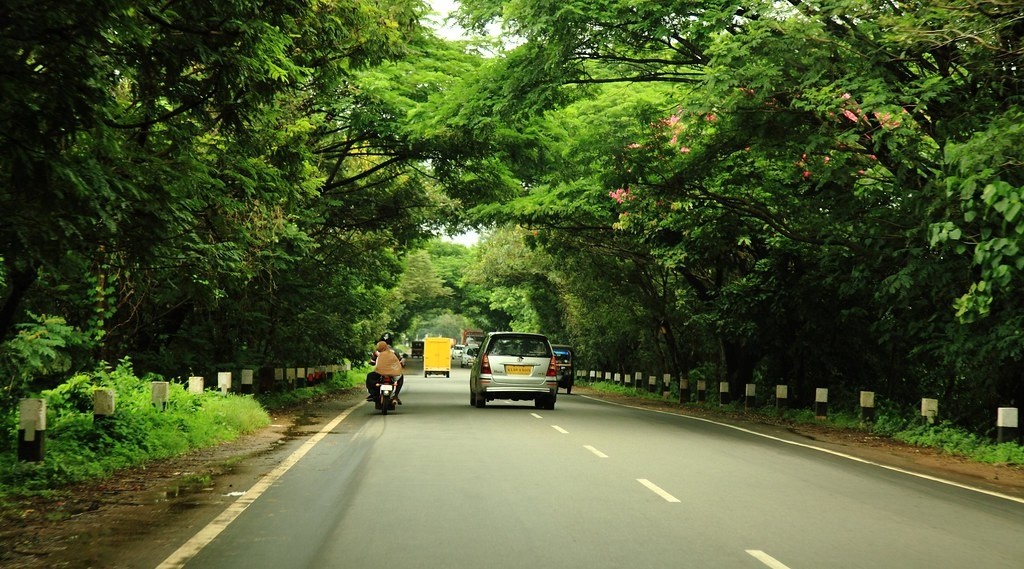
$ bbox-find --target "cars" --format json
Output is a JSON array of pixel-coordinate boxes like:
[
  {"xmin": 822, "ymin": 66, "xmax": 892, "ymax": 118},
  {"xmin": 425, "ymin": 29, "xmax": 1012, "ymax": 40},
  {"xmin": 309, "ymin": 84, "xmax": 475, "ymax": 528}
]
[
  {"xmin": 546, "ymin": 343, "xmax": 576, "ymax": 394},
  {"xmin": 467, "ymin": 330, "xmax": 562, "ymax": 410},
  {"xmin": 451, "ymin": 344, "xmax": 466, "ymax": 359},
  {"xmin": 393, "ymin": 348, "xmax": 407, "ymax": 368},
  {"xmin": 460, "ymin": 344, "xmax": 481, "ymax": 368}
]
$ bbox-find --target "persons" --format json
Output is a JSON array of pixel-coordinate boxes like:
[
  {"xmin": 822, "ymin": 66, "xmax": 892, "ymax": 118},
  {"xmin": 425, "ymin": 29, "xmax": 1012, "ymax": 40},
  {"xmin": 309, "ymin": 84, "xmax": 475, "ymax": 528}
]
[{"xmin": 366, "ymin": 332, "xmax": 405, "ymax": 404}]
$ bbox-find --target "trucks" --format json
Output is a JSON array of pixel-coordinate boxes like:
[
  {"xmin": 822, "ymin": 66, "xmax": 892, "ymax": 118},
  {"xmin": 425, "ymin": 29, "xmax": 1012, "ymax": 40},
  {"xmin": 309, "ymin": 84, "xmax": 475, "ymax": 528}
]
[{"xmin": 424, "ymin": 337, "xmax": 452, "ymax": 377}]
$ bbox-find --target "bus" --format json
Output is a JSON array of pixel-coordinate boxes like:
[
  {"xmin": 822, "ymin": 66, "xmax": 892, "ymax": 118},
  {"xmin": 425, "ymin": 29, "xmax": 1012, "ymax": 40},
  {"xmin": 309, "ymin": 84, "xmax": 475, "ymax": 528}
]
[{"xmin": 461, "ymin": 329, "xmax": 486, "ymax": 351}]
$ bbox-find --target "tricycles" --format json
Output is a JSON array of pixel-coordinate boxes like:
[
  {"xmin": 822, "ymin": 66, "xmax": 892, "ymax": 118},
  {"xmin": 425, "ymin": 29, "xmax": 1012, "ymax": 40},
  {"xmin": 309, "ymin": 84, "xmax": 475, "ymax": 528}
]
[{"xmin": 411, "ymin": 341, "xmax": 424, "ymax": 359}]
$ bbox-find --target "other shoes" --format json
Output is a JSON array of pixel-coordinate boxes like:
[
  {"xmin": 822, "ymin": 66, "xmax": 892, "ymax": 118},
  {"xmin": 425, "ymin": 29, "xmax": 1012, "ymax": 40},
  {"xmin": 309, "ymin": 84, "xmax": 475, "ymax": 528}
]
[
  {"xmin": 366, "ymin": 395, "xmax": 375, "ymax": 402},
  {"xmin": 393, "ymin": 396, "xmax": 401, "ymax": 406}
]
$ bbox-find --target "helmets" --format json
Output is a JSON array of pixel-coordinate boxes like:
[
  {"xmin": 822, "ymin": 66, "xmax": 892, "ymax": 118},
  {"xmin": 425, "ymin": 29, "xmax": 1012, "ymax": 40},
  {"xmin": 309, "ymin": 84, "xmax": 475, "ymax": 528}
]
[{"xmin": 379, "ymin": 333, "xmax": 393, "ymax": 346}]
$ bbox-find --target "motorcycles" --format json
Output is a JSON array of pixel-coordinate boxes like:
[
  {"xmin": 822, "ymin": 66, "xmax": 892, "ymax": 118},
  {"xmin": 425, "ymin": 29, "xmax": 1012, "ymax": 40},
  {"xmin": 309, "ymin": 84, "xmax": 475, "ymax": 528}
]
[{"xmin": 375, "ymin": 352, "xmax": 409, "ymax": 416}]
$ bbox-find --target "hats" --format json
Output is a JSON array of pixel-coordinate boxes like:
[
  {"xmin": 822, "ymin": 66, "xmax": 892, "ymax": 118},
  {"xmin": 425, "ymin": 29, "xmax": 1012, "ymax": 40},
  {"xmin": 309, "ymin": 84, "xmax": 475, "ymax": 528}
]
[{"xmin": 377, "ymin": 341, "xmax": 387, "ymax": 351}]
